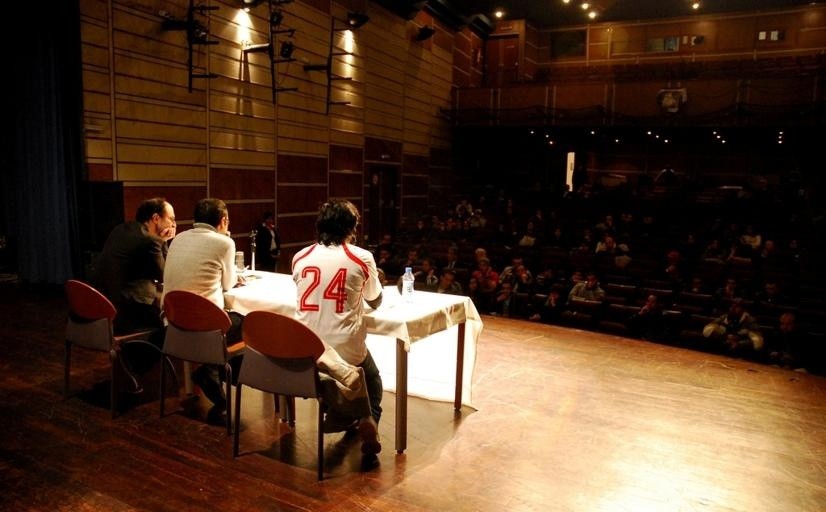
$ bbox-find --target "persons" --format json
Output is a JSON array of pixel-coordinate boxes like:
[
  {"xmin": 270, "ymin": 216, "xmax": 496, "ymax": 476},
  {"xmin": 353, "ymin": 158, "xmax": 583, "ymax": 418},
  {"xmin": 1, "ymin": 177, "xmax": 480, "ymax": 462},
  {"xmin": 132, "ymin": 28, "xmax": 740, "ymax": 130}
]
[
  {"xmin": 160, "ymin": 197, "xmax": 247, "ymax": 409},
  {"xmin": 289, "ymin": 196, "xmax": 385, "ymax": 455},
  {"xmin": 254, "ymin": 208, "xmax": 282, "ymax": 272},
  {"xmin": 351, "ymin": 153, "xmax": 826, "ymax": 375},
  {"xmin": 91, "ymin": 196, "xmax": 182, "ymax": 395}
]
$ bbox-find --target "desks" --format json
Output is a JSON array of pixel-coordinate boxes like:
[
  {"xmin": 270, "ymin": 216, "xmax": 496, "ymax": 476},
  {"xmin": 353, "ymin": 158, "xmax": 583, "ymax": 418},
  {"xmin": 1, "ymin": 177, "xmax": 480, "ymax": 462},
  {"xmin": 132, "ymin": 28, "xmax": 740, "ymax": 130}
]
[{"xmin": 223, "ymin": 265, "xmax": 467, "ymax": 454}]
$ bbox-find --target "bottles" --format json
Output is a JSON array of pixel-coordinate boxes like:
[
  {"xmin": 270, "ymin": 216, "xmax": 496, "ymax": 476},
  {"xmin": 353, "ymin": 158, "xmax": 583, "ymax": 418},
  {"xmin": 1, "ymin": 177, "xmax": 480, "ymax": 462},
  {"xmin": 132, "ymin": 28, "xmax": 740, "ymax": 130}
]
[{"xmin": 402, "ymin": 267, "xmax": 416, "ymax": 303}]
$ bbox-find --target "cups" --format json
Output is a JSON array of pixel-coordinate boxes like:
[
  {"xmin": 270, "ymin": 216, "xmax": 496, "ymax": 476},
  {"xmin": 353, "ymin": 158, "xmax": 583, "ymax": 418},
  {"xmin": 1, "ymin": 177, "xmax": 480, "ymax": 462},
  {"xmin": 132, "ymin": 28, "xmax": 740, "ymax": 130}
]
[{"xmin": 235, "ymin": 251, "xmax": 244, "ymax": 270}]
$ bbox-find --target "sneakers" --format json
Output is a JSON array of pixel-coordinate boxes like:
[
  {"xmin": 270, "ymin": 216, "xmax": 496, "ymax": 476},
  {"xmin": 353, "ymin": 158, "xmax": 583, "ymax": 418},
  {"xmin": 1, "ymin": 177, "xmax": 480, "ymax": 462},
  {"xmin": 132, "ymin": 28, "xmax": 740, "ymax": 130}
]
[
  {"xmin": 359, "ymin": 416, "xmax": 381, "ymax": 454},
  {"xmin": 362, "ymin": 441, "xmax": 380, "ymax": 472},
  {"xmin": 111, "ymin": 350, "xmax": 143, "ymax": 394},
  {"xmin": 191, "ymin": 366, "xmax": 226, "ymax": 410}
]
